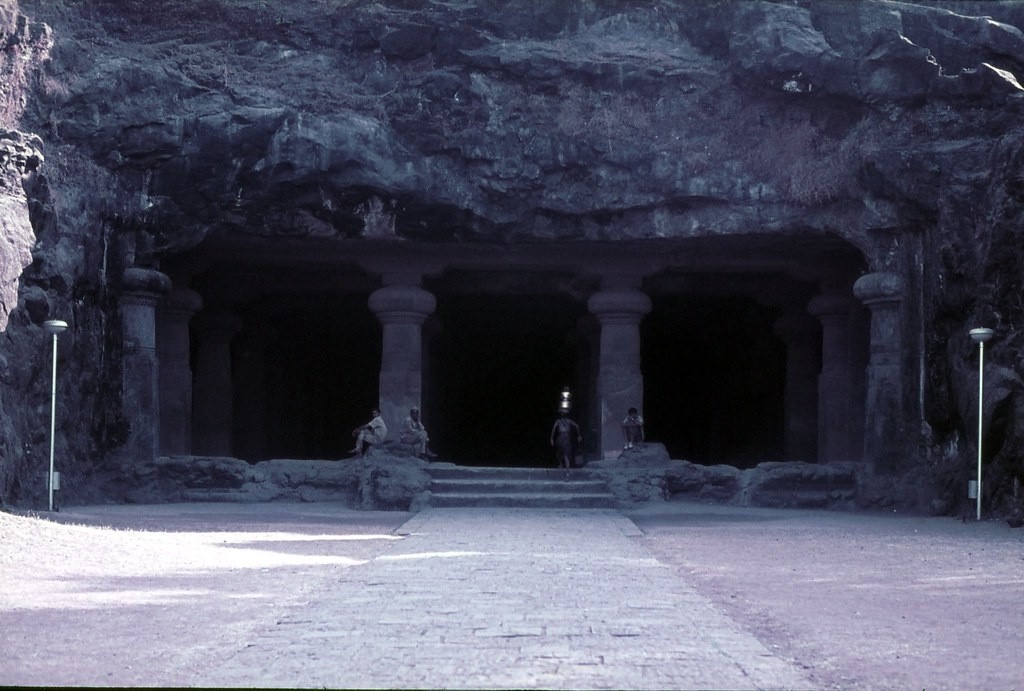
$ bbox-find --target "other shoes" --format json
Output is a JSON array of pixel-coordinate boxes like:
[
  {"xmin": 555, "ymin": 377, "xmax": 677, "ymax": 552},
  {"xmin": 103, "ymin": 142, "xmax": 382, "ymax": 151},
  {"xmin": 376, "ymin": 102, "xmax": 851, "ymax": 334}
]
[
  {"xmin": 624, "ymin": 442, "xmax": 634, "ymax": 449},
  {"xmin": 425, "ymin": 450, "xmax": 438, "ymax": 458},
  {"xmin": 419, "ymin": 454, "xmax": 427, "ymax": 462},
  {"xmin": 349, "ymin": 450, "xmax": 362, "ymax": 456}
]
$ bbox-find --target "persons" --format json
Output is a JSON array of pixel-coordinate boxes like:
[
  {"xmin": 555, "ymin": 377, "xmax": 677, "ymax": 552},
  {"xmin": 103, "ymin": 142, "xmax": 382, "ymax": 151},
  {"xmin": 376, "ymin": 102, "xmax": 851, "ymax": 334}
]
[
  {"xmin": 550, "ymin": 411, "xmax": 582, "ymax": 482},
  {"xmin": 349, "ymin": 409, "xmax": 387, "ymax": 457},
  {"xmin": 400, "ymin": 407, "xmax": 437, "ymax": 461},
  {"xmin": 622, "ymin": 408, "xmax": 645, "ymax": 450}
]
